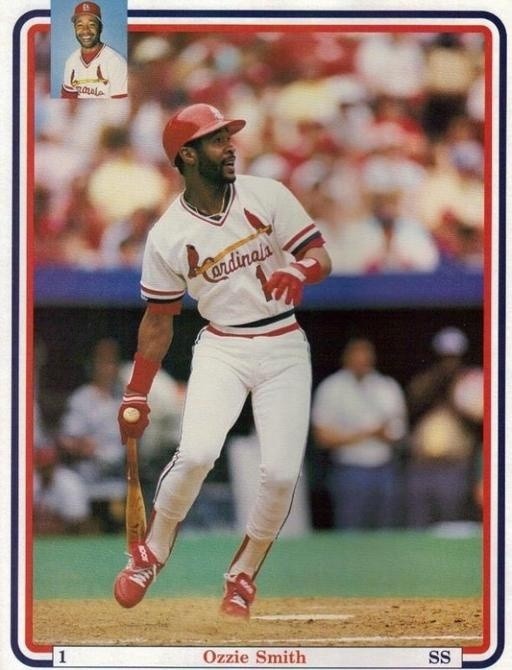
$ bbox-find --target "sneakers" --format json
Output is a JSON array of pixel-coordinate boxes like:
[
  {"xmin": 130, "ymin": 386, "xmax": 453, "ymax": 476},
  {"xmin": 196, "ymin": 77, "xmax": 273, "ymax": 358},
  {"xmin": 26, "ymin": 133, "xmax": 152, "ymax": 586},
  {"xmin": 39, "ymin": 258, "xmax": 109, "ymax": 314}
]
[
  {"xmin": 220, "ymin": 573, "xmax": 258, "ymax": 618},
  {"xmin": 115, "ymin": 542, "xmax": 164, "ymax": 609}
]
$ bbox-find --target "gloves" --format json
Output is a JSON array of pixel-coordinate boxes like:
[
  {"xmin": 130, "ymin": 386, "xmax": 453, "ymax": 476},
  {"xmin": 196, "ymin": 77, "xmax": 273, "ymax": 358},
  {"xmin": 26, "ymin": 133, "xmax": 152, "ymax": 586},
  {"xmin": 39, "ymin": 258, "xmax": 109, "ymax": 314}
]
[
  {"xmin": 262, "ymin": 263, "xmax": 309, "ymax": 308},
  {"xmin": 118, "ymin": 393, "xmax": 153, "ymax": 444}
]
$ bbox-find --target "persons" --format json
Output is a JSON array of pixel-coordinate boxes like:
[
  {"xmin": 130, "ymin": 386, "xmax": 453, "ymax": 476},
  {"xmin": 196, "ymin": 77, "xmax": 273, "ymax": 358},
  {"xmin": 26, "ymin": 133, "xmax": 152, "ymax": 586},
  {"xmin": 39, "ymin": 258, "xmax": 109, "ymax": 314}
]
[
  {"xmin": 58, "ymin": 1, "xmax": 129, "ymax": 100},
  {"xmin": 112, "ymin": 102, "xmax": 333, "ymax": 625},
  {"xmin": 29, "ymin": 23, "xmax": 486, "ymax": 535}
]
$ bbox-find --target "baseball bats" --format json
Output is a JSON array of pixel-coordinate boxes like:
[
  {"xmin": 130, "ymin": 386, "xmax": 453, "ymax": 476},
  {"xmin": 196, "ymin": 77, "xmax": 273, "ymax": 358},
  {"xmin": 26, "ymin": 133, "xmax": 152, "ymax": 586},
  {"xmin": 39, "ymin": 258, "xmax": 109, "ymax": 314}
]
[{"xmin": 123, "ymin": 407, "xmax": 147, "ymax": 555}]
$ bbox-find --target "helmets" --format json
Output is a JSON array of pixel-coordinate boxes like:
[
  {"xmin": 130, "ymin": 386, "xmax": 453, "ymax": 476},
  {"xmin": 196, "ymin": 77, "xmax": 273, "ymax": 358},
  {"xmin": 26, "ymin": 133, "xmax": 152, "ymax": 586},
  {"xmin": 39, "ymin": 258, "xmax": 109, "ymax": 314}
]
[
  {"xmin": 161, "ymin": 103, "xmax": 246, "ymax": 166},
  {"xmin": 70, "ymin": 2, "xmax": 103, "ymax": 24}
]
